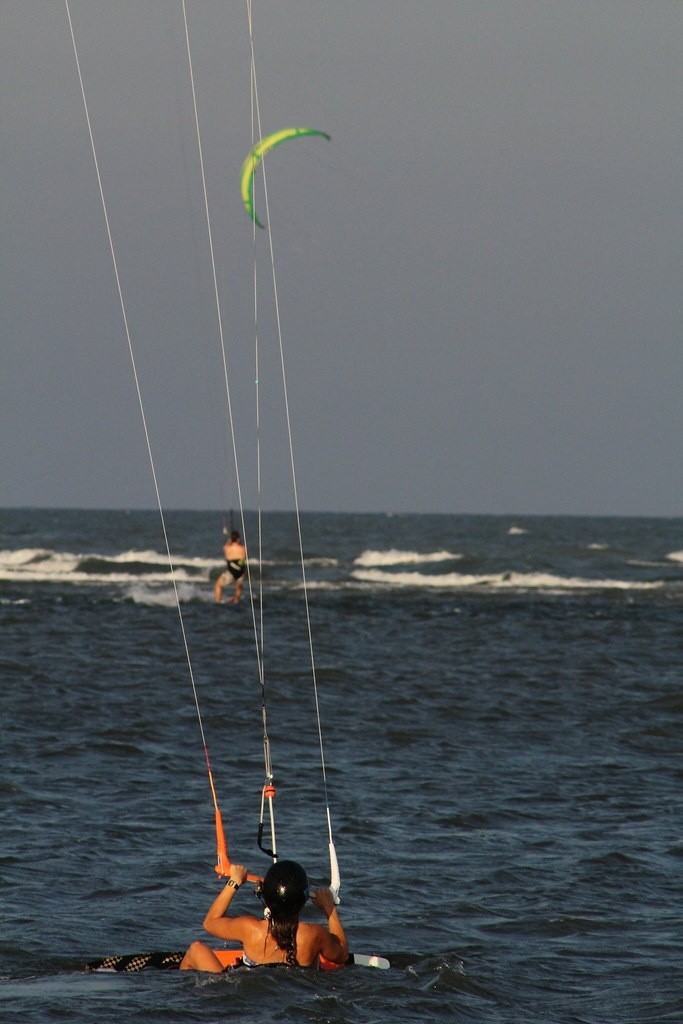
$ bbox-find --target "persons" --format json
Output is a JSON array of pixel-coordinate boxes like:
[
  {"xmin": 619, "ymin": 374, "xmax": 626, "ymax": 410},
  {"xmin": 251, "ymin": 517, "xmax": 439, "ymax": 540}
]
[
  {"xmin": 179, "ymin": 860, "xmax": 348, "ymax": 973},
  {"xmin": 214, "ymin": 531, "xmax": 246, "ymax": 603}
]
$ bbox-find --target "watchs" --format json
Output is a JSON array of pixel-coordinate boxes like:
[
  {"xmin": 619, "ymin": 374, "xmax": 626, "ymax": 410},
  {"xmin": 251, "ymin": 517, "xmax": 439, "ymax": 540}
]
[{"xmin": 226, "ymin": 880, "xmax": 240, "ymax": 891}]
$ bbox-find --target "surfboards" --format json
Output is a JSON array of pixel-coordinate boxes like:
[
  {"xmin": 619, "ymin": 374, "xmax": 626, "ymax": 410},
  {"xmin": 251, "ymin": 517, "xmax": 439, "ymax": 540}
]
[{"xmin": 82, "ymin": 952, "xmax": 391, "ymax": 973}]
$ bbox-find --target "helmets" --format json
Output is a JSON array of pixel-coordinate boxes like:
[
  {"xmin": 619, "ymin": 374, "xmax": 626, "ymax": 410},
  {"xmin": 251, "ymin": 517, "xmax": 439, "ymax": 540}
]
[{"xmin": 262, "ymin": 861, "xmax": 310, "ymax": 916}]
